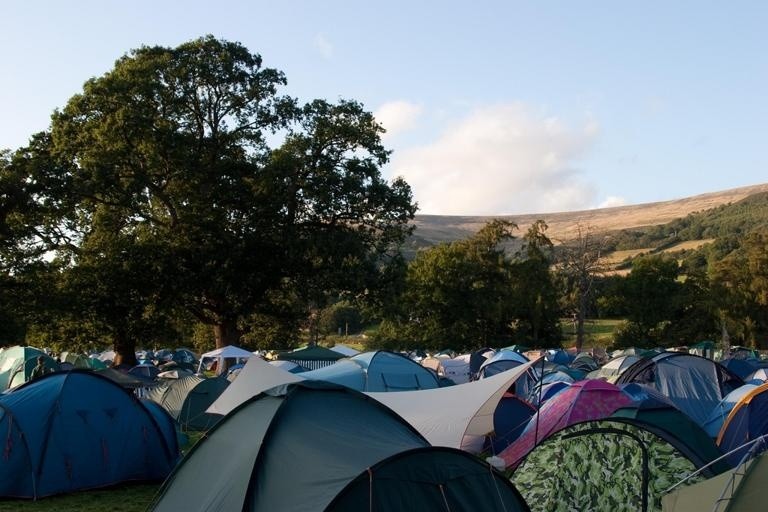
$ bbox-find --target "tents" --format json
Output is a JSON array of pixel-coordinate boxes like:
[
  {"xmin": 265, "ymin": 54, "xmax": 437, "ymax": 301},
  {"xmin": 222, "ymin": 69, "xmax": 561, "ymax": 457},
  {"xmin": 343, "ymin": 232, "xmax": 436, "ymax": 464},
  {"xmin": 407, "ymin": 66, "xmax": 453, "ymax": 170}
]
[{"xmin": 1, "ymin": 340, "xmax": 768, "ymax": 511}]
